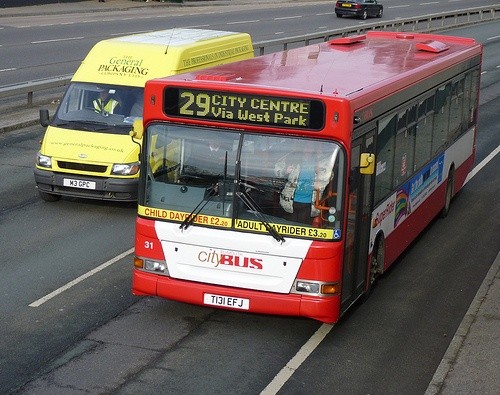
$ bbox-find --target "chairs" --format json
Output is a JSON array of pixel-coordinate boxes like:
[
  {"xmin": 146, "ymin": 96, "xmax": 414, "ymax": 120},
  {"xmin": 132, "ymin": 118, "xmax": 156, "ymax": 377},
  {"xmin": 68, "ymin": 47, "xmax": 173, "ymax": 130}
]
[{"xmin": 129, "ymin": 93, "xmax": 143, "ymax": 117}]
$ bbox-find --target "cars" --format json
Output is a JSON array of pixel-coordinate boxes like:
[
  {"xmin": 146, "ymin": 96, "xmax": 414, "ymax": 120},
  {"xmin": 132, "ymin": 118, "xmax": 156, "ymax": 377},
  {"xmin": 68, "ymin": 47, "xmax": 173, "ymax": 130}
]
[{"xmin": 335, "ymin": 0, "xmax": 384, "ymax": 21}]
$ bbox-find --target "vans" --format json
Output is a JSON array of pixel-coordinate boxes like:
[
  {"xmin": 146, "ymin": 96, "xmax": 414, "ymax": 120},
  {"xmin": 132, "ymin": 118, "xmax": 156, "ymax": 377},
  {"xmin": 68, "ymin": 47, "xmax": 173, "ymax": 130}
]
[{"xmin": 34, "ymin": 27, "xmax": 256, "ymax": 205}]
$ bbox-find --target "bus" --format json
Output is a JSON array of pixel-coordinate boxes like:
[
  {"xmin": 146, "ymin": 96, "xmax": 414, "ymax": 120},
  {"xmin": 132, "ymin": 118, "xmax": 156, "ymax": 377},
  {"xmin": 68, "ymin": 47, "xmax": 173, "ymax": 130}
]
[{"xmin": 130, "ymin": 31, "xmax": 484, "ymax": 325}]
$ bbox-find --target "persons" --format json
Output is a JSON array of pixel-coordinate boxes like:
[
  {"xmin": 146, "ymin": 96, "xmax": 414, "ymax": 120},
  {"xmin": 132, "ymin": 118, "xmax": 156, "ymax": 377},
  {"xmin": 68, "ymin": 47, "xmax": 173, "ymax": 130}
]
[
  {"xmin": 90, "ymin": 90, "xmax": 122, "ymax": 114},
  {"xmin": 179, "ymin": 130, "xmax": 333, "ymax": 223}
]
